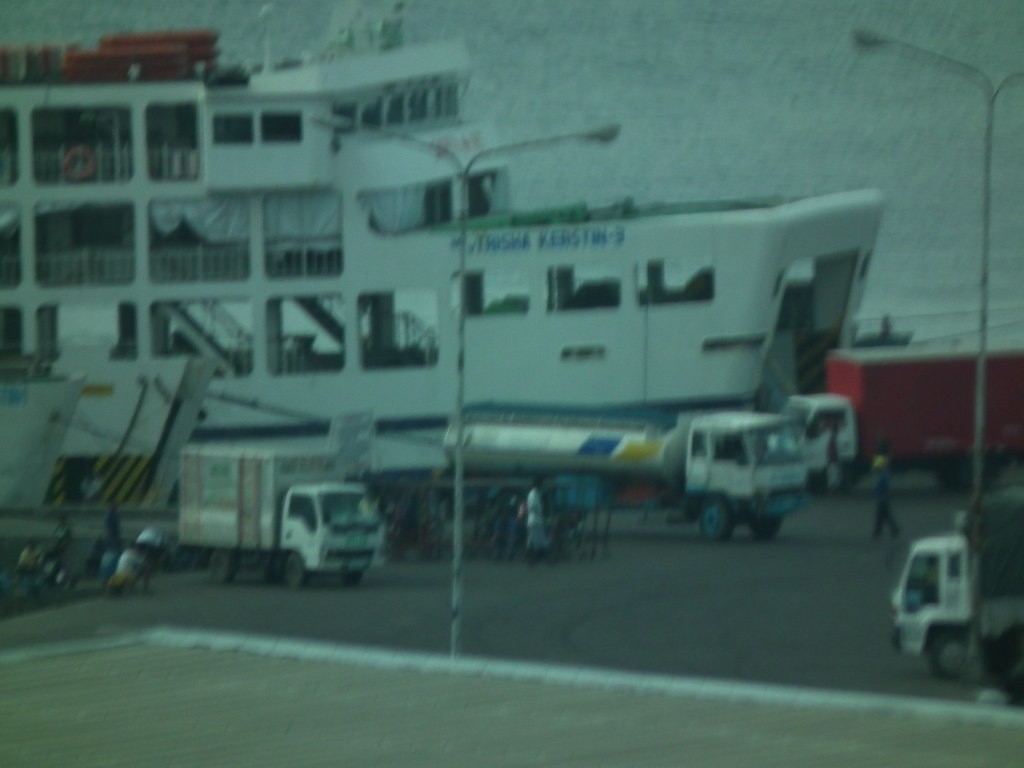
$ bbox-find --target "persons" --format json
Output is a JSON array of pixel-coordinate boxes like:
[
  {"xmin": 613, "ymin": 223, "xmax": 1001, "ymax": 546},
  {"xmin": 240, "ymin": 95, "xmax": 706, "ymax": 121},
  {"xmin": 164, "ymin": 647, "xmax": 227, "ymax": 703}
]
[
  {"xmin": 18, "ymin": 539, "xmax": 45, "ymax": 580},
  {"xmin": 105, "ymin": 525, "xmax": 163, "ymax": 597},
  {"xmin": 489, "ymin": 493, "xmax": 526, "ymax": 559},
  {"xmin": 875, "ymin": 471, "xmax": 900, "ymax": 540},
  {"xmin": 524, "ymin": 481, "xmax": 554, "ymax": 564}
]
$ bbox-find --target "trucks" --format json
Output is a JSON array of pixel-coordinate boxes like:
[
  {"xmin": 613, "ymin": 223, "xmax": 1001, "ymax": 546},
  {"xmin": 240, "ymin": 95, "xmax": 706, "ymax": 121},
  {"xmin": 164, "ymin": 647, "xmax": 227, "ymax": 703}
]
[
  {"xmin": 436, "ymin": 406, "xmax": 810, "ymax": 543},
  {"xmin": 176, "ymin": 435, "xmax": 387, "ymax": 591},
  {"xmin": 778, "ymin": 342, "xmax": 1024, "ymax": 495},
  {"xmin": 890, "ymin": 494, "xmax": 1023, "ymax": 704}
]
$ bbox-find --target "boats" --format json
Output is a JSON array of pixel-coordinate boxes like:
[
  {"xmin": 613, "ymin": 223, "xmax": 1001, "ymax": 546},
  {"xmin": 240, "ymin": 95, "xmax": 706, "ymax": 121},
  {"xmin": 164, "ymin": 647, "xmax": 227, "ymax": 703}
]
[{"xmin": 0, "ymin": 19, "xmax": 889, "ymax": 601}]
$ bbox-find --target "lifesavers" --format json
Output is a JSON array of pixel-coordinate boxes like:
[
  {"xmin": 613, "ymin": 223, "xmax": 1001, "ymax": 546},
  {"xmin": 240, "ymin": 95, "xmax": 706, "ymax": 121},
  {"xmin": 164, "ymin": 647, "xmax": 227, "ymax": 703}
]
[{"xmin": 64, "ymin": 146, "xmax": 97, "ymax": 179}]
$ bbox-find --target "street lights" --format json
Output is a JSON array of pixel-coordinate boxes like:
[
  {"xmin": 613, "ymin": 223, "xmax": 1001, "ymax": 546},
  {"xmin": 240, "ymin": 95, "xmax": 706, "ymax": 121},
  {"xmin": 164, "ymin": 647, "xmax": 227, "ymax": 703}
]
[
  {"xmin": 846, "ymin": 23, "xmax": 1023, "ymax": 536},
  {"xmin": 312, "ymin": 104, "xmax": 623, "ymax": 661}
]
